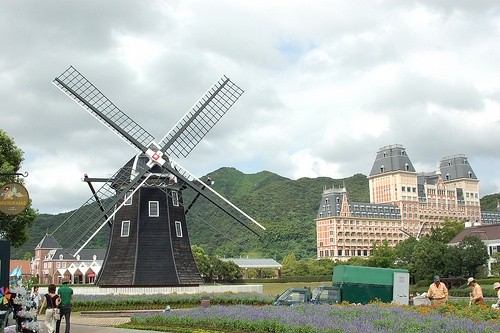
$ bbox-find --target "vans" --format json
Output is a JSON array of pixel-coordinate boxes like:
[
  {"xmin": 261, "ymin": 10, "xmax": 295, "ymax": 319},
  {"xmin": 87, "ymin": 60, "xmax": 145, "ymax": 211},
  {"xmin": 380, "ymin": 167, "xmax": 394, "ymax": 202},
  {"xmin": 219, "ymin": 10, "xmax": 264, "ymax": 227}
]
[{"xmin": 273, "ymin": 286, "xmax": 311, "ymax": 306}]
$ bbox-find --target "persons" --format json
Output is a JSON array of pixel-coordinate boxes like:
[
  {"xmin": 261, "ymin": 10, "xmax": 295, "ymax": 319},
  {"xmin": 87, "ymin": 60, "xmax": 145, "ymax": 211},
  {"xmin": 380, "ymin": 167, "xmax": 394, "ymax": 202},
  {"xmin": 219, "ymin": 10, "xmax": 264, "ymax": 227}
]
[
  {"xmin": 31, "ymin": 286, "xmax": 43, "ymax": 313},
  {"xmin": 427, "ymin": 275, "xmax": 449, "ymax": 305},
  {"xmin": 467, "ymin": 277, "xmax": 484, "ymax": 305},
  {"xmin": 493, "ymin": 282, "xmax": 500, "ymax": 307},
  {"xmin": 39, "ymin": 284, "xmax": 60, "ymax": 333},
  {"xmin": 56, "ymin": 278, "xmax": 73, "ymax": 333}
]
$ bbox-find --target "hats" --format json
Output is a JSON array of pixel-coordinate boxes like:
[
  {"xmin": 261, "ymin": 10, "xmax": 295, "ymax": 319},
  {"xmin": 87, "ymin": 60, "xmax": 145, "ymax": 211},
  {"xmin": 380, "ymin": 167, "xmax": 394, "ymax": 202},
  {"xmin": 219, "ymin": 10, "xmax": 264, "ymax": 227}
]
[
  {"xmin": 434, "ymin": 275, "xmax": 440, "ymax": 281},
  {"xmin": 62, "ymin": 278, "xmax": 69, "ymax": 283},
  {"xmin": 493, "ymin": 282, "xmax": 500, "ymax": 289},
  {"xmin": 467, "ymin": 278, "xmax": 474, "ymax": 285}
]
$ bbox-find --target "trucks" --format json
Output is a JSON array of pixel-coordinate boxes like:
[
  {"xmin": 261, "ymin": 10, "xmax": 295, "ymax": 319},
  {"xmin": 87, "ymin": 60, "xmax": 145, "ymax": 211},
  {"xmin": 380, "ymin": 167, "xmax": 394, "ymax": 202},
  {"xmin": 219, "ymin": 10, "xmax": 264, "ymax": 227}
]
[{"xmin": 272, "ymin": 265, "xmax": 409, "ymax": 307}]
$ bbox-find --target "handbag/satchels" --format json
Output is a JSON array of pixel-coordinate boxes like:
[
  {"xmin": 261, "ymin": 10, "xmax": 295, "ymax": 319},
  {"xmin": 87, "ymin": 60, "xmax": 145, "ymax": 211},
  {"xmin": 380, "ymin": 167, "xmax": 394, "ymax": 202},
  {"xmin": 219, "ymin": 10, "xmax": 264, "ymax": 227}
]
[{"xmin": 53, "ymin": 311, "xmax": 61, "ymax": 320}]
